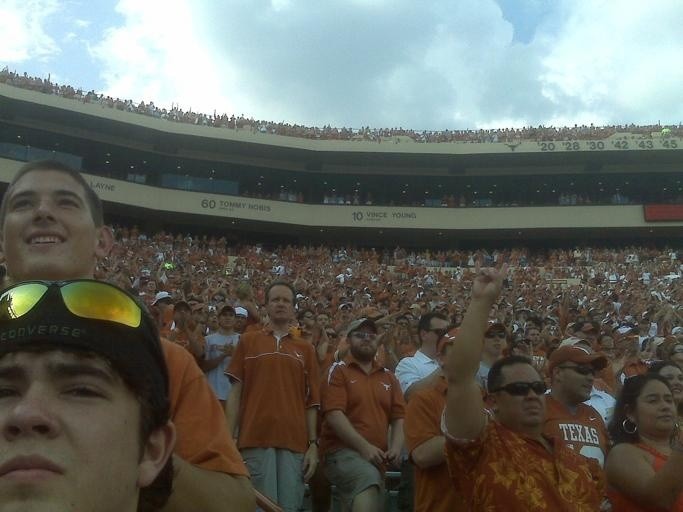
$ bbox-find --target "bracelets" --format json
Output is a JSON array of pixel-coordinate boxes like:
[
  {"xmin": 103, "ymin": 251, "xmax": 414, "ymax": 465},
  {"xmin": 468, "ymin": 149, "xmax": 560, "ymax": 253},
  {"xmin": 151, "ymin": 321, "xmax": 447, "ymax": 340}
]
[{"xmin": 306, "ymin": 439, "xmax": 319, "ymax": 448}]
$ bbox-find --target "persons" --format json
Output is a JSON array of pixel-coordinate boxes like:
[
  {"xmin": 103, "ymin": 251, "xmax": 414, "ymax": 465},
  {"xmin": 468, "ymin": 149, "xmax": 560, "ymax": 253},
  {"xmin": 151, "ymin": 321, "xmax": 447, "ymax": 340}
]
[
  {"xmin": 225, "ymin": 239, "xmax": 682, "ymax": 512},
  {"xmin": 129, "ymin": 160, "xmax": 680, "ymax": 208},
  {"xmin": 0, "ymin": 160, "xmax": 257, "ymax": 512},
  {"xmin": 1, "ymin": 66, "xmax": 683, "ymax": 143}
]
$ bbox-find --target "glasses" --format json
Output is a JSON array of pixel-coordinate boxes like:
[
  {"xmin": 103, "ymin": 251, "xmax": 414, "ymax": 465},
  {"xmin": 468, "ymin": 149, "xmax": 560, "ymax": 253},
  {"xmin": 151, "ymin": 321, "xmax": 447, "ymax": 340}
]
[
  {"xmin": 0, "ymin": 278, "xmax": 155, "ymax": 345},
  {"xmin": 558, "ymin": 364, "xmax": 596, "ymax": 377},
  {"xmin": 492, "ymin": 380, "xmax": 547, "ymax": 397}
]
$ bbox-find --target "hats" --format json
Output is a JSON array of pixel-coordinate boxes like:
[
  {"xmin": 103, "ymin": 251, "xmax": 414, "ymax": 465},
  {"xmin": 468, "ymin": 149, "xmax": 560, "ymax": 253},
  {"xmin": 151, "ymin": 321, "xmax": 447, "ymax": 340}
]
[
  {"xmin": 151, "ymin": 266, "xmax": 683, "ymax": 353},
  {"xmin": 547, "ymin": 345, "xmax": 610, "ymax": 377}
]
